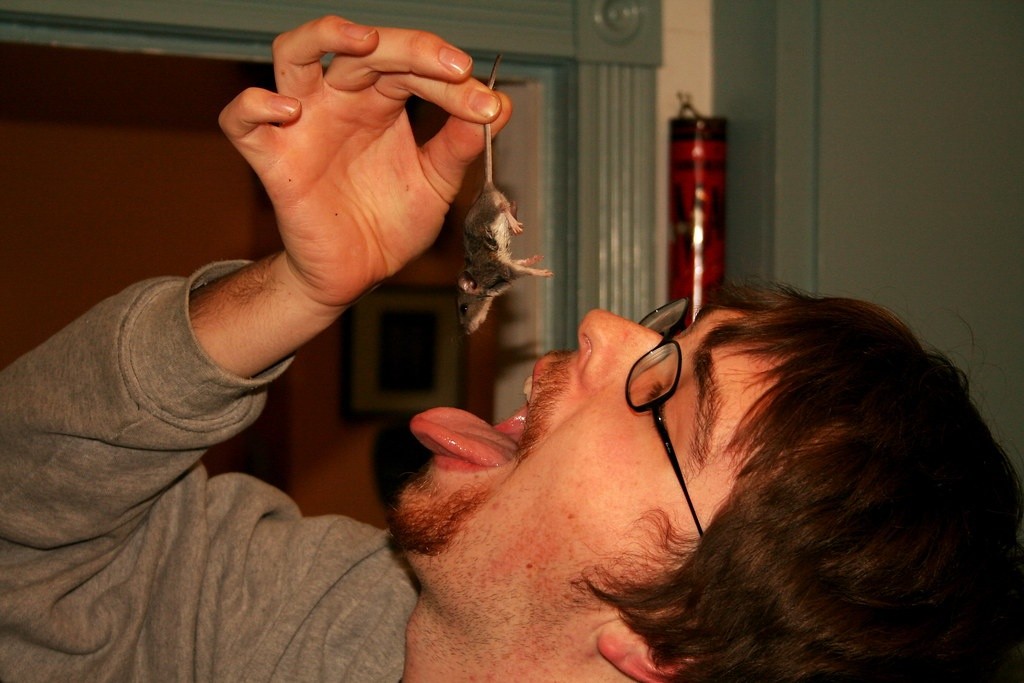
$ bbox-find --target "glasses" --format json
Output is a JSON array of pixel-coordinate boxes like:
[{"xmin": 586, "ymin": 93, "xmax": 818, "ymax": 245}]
[{"xmin": 625, "ymin": 298, "xmax": 703, "ymax": 535}]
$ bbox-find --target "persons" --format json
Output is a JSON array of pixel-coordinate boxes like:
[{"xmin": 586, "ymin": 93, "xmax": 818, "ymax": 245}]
[{"xmin": 0, "ymin": 14, "xmax": 1024, "ymax": 683}]
